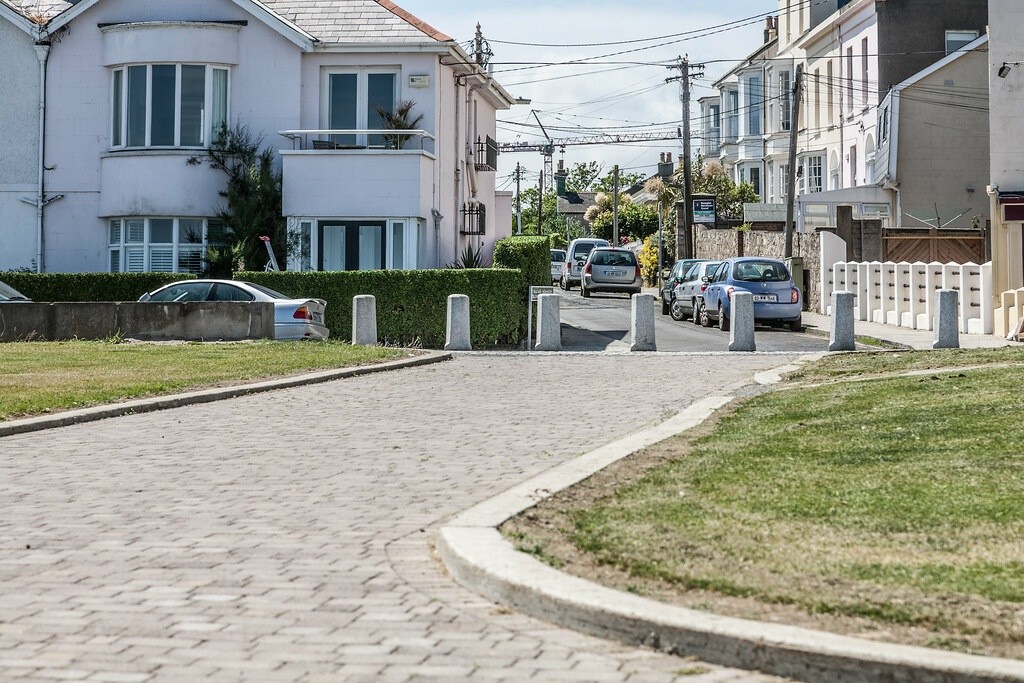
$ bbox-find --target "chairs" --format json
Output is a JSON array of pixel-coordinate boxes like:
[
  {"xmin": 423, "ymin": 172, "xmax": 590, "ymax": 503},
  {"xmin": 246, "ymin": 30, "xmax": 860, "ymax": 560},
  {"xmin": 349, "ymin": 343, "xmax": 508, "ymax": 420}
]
[
  {"xmin": 595, "ymin": 255, "xmax": 606, "ymax": 265},
  {"xmin": 613, "ymin": 257, "xmax": 628, "ymax": 267},
  {"xmin": 607, "ymin": 259, "xmax": 616, "ymax": 267},
  {"xmin": 760, "ymin": 269, "xmax": 778, "ymax": 280}
]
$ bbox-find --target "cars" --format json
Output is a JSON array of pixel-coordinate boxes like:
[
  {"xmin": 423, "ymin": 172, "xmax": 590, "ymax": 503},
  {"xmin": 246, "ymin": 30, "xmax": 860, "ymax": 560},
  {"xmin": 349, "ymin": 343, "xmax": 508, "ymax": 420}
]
[
  {"xmin": 548, "ymin": 249, "xmax": 566, "ymax": 286},
  {"xmin": 0, "ymin": 280, "xmax": 34, "ymax": 304},
  {"xmin": 699, "ymin": 256, "xmax": 802, "ymax": 333},
  {"xmin": 136, "ymin": 279, "xmax": 331, "ymax": 343}
]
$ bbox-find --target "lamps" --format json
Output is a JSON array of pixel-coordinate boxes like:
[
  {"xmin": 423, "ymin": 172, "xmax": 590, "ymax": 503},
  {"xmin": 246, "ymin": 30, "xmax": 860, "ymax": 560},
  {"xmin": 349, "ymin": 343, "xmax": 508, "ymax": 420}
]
[{"xmin": 997, "ymin": 62, "xmax": 1012, "ymax": 78}]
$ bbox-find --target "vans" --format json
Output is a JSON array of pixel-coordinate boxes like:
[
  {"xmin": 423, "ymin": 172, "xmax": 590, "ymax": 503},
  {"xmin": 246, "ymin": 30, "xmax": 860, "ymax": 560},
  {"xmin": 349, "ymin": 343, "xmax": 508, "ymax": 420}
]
[{"xmin": 559, "ymin": 237, "xmax": 611, "ymax": 291}]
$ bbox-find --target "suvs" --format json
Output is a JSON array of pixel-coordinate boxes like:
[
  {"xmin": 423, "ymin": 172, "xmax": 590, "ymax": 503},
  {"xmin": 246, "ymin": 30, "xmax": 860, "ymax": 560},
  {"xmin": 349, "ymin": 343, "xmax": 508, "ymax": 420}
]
[
  {"xmin": 661, "ymin": 259, "xmax": 717, "ymax": 315},
  {"xmin": 580, "ymin": 245, "xmax": 644, "ymax": 299},
  {"xmin": 669, "ymin": 261, "xmax": 763, "ymax": 325}
]
